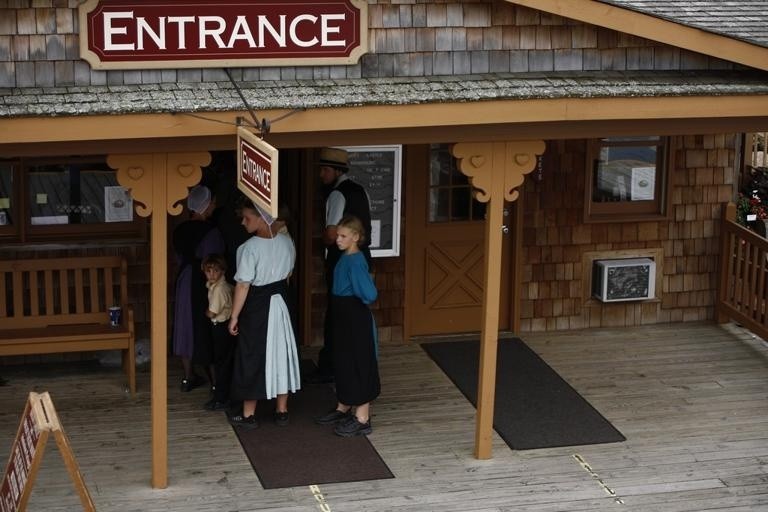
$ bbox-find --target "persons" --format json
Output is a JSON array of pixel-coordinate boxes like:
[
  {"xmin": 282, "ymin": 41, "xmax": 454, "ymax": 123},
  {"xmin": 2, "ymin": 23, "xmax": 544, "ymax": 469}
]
[
  {"xmin": 303, "ymin": 147, "xmax": 373, "ymax": 384},
  {"xmin": 168, "ymin": 183, "xmax": 231, "ymax": 395},
  {"xmin": 313, "ymin": 212, "xmax": 381, "ymax": 439},
  {"xmin": 197, "ymin": 252, "xmax": 238, "ymax": 413},
  {"xmin": 227, "ymin": 197, "xmax": 302, "ymax": 430}
]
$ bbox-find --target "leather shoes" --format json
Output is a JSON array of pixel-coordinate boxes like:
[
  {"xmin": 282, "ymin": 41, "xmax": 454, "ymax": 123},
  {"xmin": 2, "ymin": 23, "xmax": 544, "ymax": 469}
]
[
  {"xmin": 204, "ymin": 396, "xmax": 226, "ymax": 410},
  {"xmin": 181, "ymin": 379, "xmax": 192, "ymax": 391}
]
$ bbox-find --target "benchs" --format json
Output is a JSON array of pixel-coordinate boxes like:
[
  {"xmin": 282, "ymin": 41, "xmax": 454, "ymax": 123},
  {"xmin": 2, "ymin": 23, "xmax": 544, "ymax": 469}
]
[{"xmin": 0, "ymin": 256, "xmax": 138, "ymax": 394}]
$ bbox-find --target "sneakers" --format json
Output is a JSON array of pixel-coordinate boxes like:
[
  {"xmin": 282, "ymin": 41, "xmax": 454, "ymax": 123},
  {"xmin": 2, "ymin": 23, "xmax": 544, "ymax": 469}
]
[
  {"xmin": 316, "ymin": 410, "xmax": 372, "ymax": 437},
  {"xmin": 275, "ymin": 410, "xmax": 291, "ymax": 427},
  {"xmin": 229, "ymin": 415, "xmax": 260, "ymax": 431}
]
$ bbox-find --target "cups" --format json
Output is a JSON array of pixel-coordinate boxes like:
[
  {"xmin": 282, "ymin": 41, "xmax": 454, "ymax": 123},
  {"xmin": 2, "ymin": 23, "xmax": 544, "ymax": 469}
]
[{"xmin": 108, "ymin": 306, "xmax": 122, "ymax": 329}]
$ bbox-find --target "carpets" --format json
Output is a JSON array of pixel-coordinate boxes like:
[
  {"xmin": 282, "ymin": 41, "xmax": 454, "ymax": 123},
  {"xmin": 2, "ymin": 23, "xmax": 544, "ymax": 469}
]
[
  {"xmin": 420, "ymin": 337, "xmax": 627, "ymax": 451},
  {"xmin": 226, "ymin": 357, "xmax": 396, "ymax": 489}
]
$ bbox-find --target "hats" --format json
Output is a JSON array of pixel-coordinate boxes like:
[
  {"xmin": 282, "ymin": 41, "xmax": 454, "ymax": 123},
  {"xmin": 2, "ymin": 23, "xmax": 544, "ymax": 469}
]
[
  {"xmin": 187, "ymin": 184, "xmax": 212, "ymax": 214},
  {"xmin": 313, "ymin": 147, "xmax": 349, "ymax": 173}
]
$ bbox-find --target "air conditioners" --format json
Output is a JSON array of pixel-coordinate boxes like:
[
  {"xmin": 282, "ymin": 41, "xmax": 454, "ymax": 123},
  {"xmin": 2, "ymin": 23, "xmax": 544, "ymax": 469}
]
[{"xmin": 593, "ymin": 258, "xmax": 657, "ymax": 303}]
